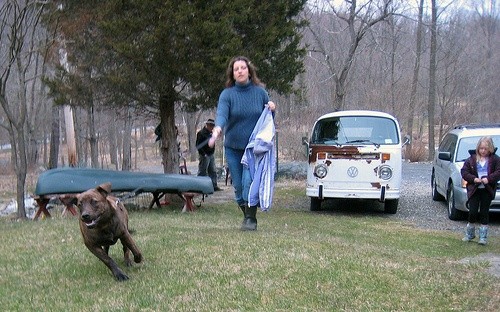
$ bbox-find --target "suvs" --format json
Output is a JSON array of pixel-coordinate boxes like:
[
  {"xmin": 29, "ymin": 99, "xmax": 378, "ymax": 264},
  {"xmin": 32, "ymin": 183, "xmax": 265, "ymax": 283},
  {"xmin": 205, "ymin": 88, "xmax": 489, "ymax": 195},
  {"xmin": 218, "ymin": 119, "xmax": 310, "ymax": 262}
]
[{"xmin": 430, "ymin": 123, "xmax": 500, "ymax": 220}]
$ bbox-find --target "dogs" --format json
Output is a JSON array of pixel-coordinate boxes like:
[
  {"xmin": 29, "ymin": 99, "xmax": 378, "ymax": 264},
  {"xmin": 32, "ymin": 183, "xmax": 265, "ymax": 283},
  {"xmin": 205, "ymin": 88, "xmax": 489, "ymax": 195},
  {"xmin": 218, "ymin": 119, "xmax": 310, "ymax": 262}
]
[{"xmin": 59, "ymin": 182, "xmax": 143, "ymax": 282}]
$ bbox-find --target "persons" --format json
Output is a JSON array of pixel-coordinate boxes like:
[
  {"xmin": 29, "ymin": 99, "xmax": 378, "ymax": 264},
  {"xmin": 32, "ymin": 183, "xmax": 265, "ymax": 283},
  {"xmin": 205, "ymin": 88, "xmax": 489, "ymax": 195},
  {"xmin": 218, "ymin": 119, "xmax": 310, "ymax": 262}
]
[
  {"xmin": 461, "ymin": 136, "xmax": 500, "ymax": 245},
  {"xmin": 195, "ymin": 119, "xmax": 223, "ymax": 191},
  {"xmin": 211, "ymin": 57, "xmax": 276, "ymax": 231}
]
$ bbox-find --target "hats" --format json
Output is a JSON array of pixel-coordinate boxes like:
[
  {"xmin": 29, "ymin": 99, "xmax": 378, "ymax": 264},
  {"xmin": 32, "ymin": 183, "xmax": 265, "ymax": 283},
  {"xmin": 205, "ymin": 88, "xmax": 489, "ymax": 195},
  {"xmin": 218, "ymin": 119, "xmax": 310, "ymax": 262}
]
[{"xmin": 205, "ymin": 119, "xmax": 215, "ymax": 125}]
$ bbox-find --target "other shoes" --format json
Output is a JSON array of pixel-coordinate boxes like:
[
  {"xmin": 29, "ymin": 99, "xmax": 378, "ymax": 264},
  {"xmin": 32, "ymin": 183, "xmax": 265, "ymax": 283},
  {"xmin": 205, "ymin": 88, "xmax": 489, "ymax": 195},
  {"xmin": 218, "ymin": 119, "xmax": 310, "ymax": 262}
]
[{"xmin": 214, "ymin": 187, "xmax": 223, "ymax": 191}]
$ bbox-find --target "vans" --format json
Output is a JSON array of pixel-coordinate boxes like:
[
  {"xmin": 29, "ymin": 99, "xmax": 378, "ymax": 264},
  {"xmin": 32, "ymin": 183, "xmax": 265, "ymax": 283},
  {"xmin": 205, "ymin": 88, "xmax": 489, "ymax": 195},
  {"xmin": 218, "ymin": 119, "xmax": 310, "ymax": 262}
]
[{"xmin": 301, "ymin": 110, "xmax": 411, "ymax": 213}]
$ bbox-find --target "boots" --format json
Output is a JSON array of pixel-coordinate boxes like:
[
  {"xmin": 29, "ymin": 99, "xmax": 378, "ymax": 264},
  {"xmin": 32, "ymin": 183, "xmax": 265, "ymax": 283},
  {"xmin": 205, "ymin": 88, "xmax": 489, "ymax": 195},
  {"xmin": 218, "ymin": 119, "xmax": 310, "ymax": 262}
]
[
  {"xmin": 239, "ymin": 204, "xmax": 246, "ymax": 230},
  {"xmin": 478, "ymin": 226, "xmax": 489, "ymax": 245},
  {"xmin": 246, "ymin": 205, "xmax": 257, "ymax": 230},
  {"xmin": 462, "ymin": 225, "xmax": 475, "ymax": 241}
]
